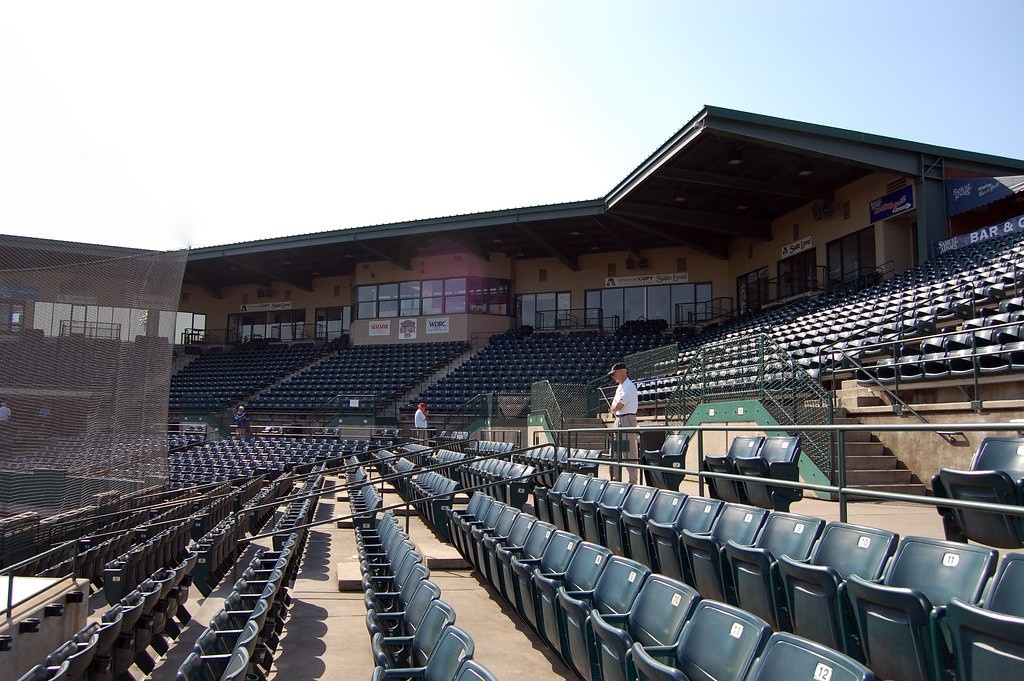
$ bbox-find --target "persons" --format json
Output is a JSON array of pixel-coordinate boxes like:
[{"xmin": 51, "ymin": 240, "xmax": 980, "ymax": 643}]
[
  {"xmin": 415, "ymin": 403, "xmax": 429, "ymax": 446},
  {"xmin": 608, "ymin": 362, "xmax": 638, "ymax": 485},
  {"xmin": 39, "ymin": 404, "xmax": 53, "ymax": 416},
  {"xmin": 0, "ymin": 402, "xmax": 12, "ymax": 421},
  {"xmin": 235, "ymin": 406, "xmax": 246, "ymax": 441}
]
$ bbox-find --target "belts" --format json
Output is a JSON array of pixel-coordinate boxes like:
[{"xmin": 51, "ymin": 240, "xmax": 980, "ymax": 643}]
[{"xmin": 615, "ymin": 413, "xmax": 635, "ymax": 417}]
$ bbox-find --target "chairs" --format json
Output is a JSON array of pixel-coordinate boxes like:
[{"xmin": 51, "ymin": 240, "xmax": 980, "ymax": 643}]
[{"xmin": 0, "ymin": 228, "xmax": 1024, "ymax": 680}]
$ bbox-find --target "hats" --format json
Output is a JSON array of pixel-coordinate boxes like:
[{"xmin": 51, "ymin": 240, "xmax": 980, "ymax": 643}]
[
  {"xmin": 607, "ymin": 362, "xmax": 627, "ymax": 375},
  {"xmin": 418, "ymin": 403, "xmax": 428, "ymax": 409}
]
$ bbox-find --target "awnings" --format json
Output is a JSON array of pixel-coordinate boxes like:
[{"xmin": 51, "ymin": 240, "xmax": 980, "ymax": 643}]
[{"xmin": 945, "ymin": 175, "xmax": 1024, "ymax": 219}]
